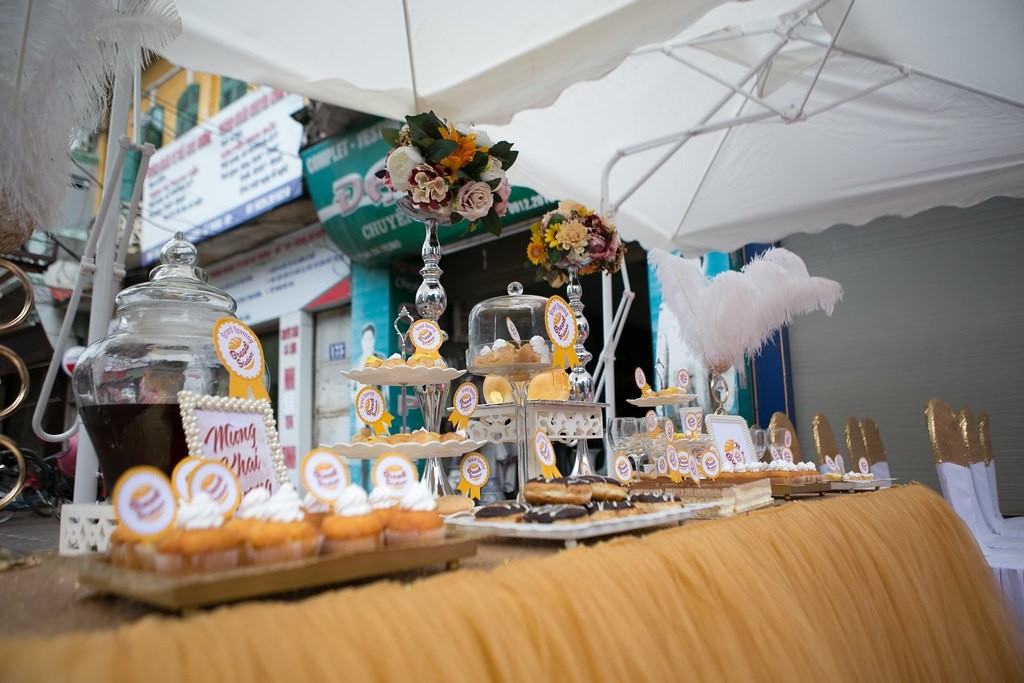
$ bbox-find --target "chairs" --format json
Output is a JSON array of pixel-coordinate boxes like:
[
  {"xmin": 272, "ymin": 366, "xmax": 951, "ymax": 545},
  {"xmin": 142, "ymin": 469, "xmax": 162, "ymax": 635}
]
[{"xmin": 751, "ymin": 396, "xmax": 1024, "ymax": 628}]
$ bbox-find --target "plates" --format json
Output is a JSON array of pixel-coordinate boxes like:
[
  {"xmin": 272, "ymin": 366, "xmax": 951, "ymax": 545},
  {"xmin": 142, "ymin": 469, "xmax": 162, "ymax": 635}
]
[
  {"xmin": 443, "ymin": 502, "xmax": 721, "ymax": 551},
  {"xmin": 770, "ymin": 481, "xmax": 830, "ymax": 499},
  {"xmin": 78, "ymin": 532, "xmax": 480, "ymax": 616},
  {"xmin": 831, "ymin": 477, "xmax": 898, "ymax": 493}
]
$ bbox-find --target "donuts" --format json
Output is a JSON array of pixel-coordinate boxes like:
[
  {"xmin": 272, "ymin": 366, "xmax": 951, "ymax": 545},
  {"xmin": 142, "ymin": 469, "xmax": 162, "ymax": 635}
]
[{"xmin": 475, "ymin": 475, "xmax": 686, "ymax": 528}]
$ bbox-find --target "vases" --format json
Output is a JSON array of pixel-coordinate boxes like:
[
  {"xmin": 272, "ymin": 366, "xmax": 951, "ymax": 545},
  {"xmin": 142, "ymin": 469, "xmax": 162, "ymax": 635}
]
[
  {"xmin": 412, "ymin": 217, "xmax": 452, "ymax": 499},
  {"xmin": 564, "ymin": 263, "xmax": 599, "ymax": 475}
]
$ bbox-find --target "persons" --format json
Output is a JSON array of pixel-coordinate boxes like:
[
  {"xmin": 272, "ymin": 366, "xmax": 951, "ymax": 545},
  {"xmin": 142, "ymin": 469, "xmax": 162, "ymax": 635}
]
[
  {"xmin": 658, "ymin": 253, "xmax": 748, "ymax": 430},
  {"xmin": 356, "ymin": 323, "xmax": 382, "ymax": 392}
]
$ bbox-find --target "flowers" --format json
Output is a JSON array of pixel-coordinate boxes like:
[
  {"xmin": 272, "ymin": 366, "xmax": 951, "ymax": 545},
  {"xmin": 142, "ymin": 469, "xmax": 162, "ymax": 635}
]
[
  {"xmin": 376, "ymin": 110, "xmax": 519, "ymax": 237},
  {"xmin": 523, "ymin": 191, "xmax": 628, "ymax": 280}
]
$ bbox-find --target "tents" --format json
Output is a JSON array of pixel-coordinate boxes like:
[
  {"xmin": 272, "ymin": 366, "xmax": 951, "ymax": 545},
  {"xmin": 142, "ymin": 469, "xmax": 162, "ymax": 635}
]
[{"xmin": 74, "ymin": 0, "xmax": 1024, "ymax": 503}]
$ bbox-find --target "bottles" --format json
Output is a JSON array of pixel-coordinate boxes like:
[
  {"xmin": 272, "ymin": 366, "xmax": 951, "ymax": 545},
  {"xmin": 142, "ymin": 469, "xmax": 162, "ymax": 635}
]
[{"xmin": 71, "ymin": 239, "xmax": 240, "ymax": 497}]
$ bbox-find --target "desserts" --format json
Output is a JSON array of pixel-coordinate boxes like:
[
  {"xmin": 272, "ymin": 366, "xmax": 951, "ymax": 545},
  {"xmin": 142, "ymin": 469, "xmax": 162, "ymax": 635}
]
[
  {"xmin": 351, "ymin": 427, "xmax": 469, "ymax": 446},
  {"xmin": 640, "ymin": 384, "xmax": 686, "ymax": 399},
  {"xmin": 364, "ymin": 351, "xmax": 438, "ymax": 368},
  {"xmin": 106, "ymin": 489, "xmax": 474, "ymax": 577},
  {"xmin": 626, "ymin": 459, "xmax": 873, "ymax": 517},
  {"xmin": 476, "ymin": 332, "xmax": 555, "ymax": 364}
]
